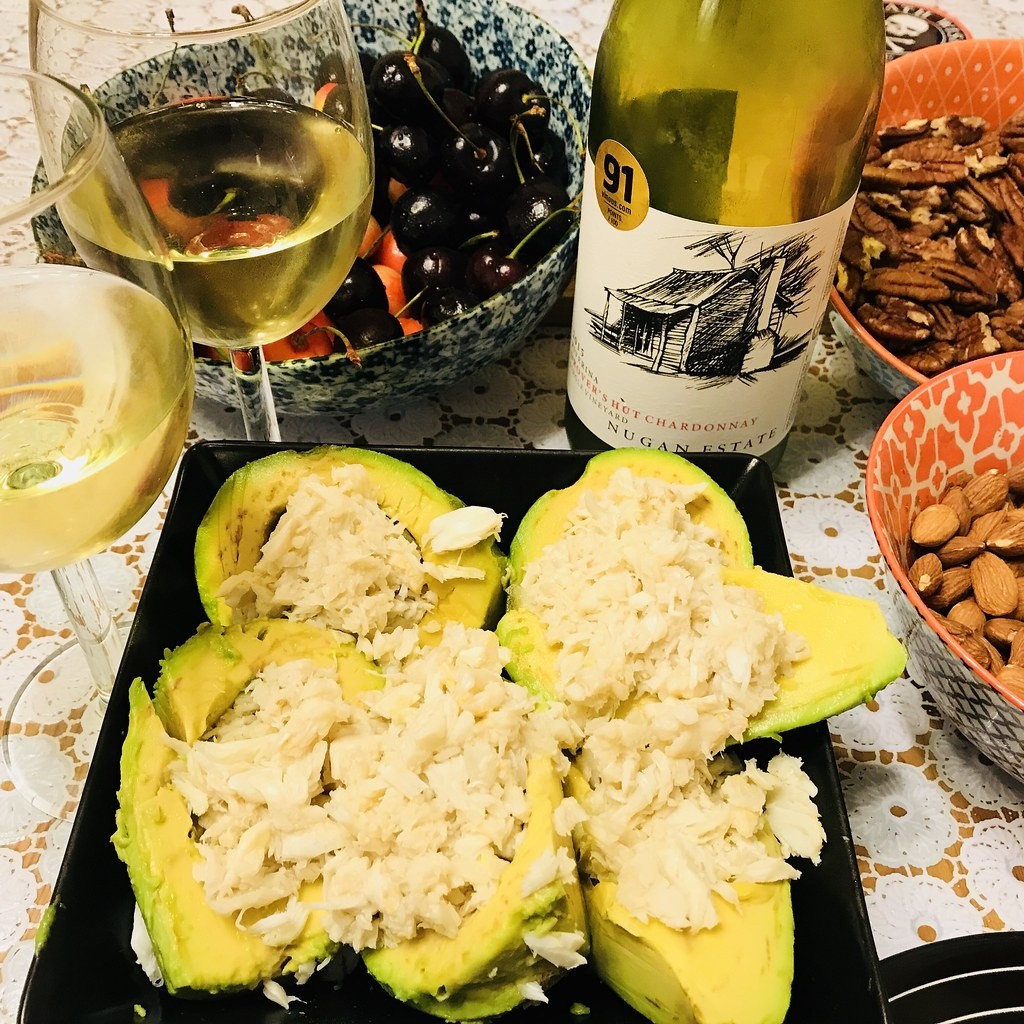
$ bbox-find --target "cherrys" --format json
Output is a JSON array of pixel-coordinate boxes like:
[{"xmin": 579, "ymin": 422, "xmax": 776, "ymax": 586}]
[{"xmin": 67, "ymin": 0, "xmax": 585, "ymax": 371}]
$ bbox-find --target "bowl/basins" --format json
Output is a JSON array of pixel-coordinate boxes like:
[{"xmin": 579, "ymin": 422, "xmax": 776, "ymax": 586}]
[
  {"xmin": 32, "ymin": 0, "xmax": 593, "ymax": 418},
  {"xmin": 827, "ymin": 37, "xmax": 1024, "ymax": 402},
  {"xmin": 864, "ymin": 349, "xmax": 1024, "ymax": 782}
]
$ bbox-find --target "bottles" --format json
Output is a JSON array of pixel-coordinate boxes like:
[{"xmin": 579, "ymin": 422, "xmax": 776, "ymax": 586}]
[{"xmin": 564, "ymin": 0, "xmax": 886, "ymax": 475}]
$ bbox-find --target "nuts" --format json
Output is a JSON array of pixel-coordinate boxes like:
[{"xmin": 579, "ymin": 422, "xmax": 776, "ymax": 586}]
[
  {"xmin": 835, "ymin": 111, "xmax": 1024, "ymax": 378},
  {"xmin": 905, "ymin": 459, "xmax": 1024, "ymax": 705}
]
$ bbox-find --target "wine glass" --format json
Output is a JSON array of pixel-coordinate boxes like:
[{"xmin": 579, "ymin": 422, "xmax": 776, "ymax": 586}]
[
  {"xmin": 0, "ymin": 64, "xmax": 195, "ymax": 823},
  {"xmin": 27, "ymin": 1, "xmax": 374, "ymax": 443}
]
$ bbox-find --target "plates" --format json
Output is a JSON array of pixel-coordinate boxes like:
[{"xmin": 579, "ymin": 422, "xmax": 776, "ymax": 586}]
[
  {"xmin": 880, "ymin": 930, "xmax": 1024, "ymax": 1024},
  {"xmin": 18, "ymin": 439, "xmax": 893, "ymax": 1024}
]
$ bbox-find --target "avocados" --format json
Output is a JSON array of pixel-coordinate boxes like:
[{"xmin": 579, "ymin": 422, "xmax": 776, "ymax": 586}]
[{"xmin": 108, "ymin": 445, "xmax": 913, "ymax": 1024}]
